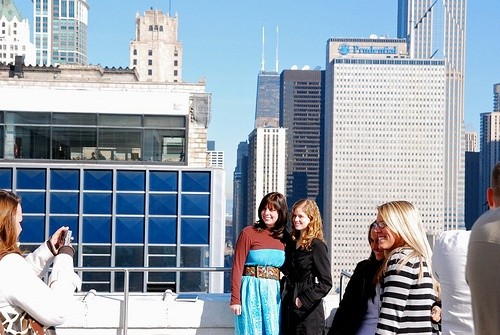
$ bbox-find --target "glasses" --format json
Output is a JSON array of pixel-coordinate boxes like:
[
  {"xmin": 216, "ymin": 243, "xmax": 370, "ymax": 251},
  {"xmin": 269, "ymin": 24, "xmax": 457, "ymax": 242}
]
[{"xmin": 372, "ymin": 221, "xmax": 386, "ymax": 229}]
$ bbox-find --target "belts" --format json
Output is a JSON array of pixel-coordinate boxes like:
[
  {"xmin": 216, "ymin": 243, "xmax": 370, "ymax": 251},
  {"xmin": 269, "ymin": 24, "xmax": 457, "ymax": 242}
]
[{"xmin": 241, "ymin": 266, "xmax": 280, "ymax": 279}]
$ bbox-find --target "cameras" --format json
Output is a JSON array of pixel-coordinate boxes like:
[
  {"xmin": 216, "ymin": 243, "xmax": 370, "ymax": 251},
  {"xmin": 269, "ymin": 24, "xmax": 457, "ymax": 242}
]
[{"xmin": 62, "ymin": 230, "xmax": 72, "ymax": 237}]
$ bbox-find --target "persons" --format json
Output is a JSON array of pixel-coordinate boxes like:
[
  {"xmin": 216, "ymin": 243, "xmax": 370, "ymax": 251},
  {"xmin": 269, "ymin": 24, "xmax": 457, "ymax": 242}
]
[
  {"xmin": 431, "ymin": 230, "xmax": 474, "ymax": 335},
  {"xmin": 0, "ymin": 190, "xmax": 75, "ymax": 335},
  {"xmin": 373, "ymin": 201, "xmax": 435, "ymax": 335},
  {"xmin": 281, "ymin": 199, "xmax": 332, "ymax": 335},
  {"xmin": 466, "ymin": 165, "xmax": 500, "ymax": 335},
  {"xmin": 327, "ymin": 220, "xmax": 441, "ymax": 335},
  {"xmin": 230, "ymin": 192, "xmax": 288, "ymax": 335}
]
